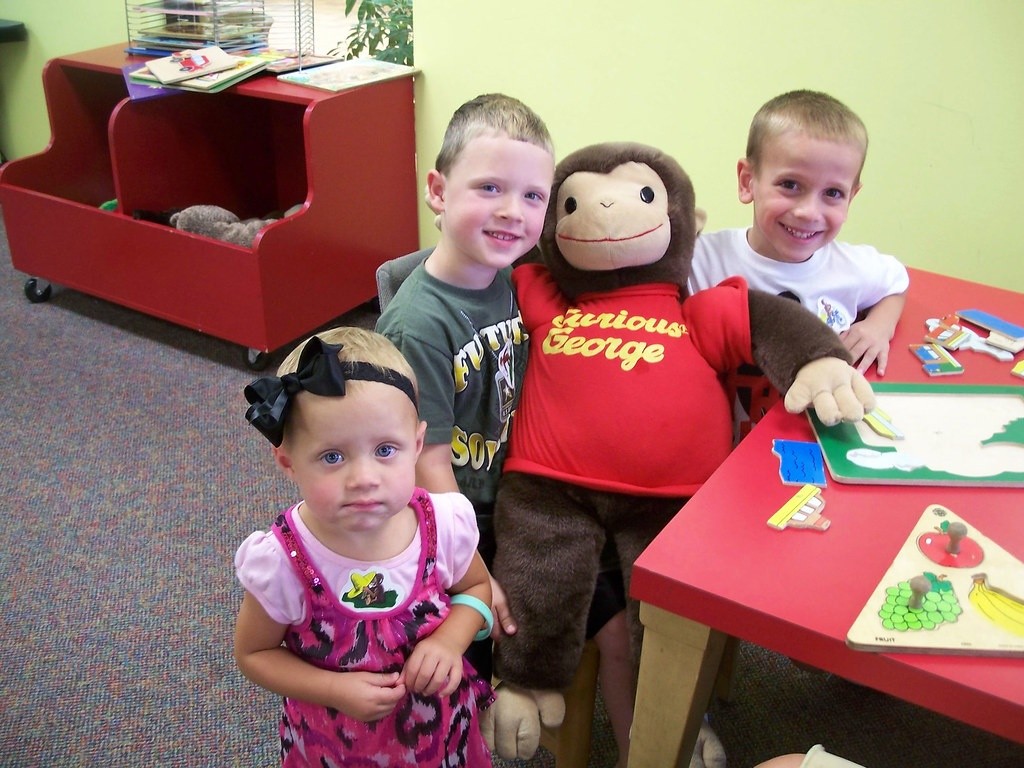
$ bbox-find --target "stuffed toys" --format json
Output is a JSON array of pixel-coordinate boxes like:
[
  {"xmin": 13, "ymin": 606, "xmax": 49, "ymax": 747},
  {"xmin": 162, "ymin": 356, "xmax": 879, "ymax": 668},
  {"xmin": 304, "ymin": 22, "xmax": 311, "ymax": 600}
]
[
  {"xmin": 169, "ymin": 205, "xmax": 303, "ymax": 249},
  {"xmin": 434, "ymin": 140, "xmax": 867, "ymax": 767}
]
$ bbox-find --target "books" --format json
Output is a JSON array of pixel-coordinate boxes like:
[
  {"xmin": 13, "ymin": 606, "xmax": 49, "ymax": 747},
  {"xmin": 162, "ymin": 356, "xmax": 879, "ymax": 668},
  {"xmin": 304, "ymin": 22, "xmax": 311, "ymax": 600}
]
[
  {"xmin": 122, "ymin": 47, "xmax": 343, "ymax": 101},
  {"xmin": 144, "ymin": 44, "xmax": 238, "ymax": 84},
  {"xmin": 277, "ymin": 58, "xmax": 423, "ymax": 93}
]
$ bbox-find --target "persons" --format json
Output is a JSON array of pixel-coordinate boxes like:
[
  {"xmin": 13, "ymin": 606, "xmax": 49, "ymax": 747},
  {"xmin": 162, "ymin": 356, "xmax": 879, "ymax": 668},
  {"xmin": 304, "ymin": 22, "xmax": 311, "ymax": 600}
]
[
  {"xmin": 232, "ymin": 326, "xmax": 493, "ymax": 768},
  {"xmin": 682, "ymin": 88, "xmax": 909, "ymax": 448},
  {"xmin": 375, "ymin": 94, "xmax": 632, "ymax": 767}
]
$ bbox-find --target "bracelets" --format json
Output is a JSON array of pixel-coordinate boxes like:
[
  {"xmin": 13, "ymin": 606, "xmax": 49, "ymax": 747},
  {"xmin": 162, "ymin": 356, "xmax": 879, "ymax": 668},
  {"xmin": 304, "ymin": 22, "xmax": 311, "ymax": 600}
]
[{"xmin": 450, "ymin": 594, "xmax": 495, "ymax": 641}]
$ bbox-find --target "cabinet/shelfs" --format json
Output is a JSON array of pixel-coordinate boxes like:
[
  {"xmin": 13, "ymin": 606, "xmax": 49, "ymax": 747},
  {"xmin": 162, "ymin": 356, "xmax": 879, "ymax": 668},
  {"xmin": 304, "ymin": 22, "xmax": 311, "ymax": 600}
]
[{"xmin": 0, "ymin": 39, "xmax": 419, "ymax": 369}]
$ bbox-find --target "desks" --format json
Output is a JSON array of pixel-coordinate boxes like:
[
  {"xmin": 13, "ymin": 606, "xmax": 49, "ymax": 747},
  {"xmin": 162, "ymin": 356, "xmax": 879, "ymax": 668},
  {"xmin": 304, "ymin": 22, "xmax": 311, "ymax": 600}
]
[{"xmin": 627, "ymin": 263, "xmax": 1024, "ymax": 768}]
